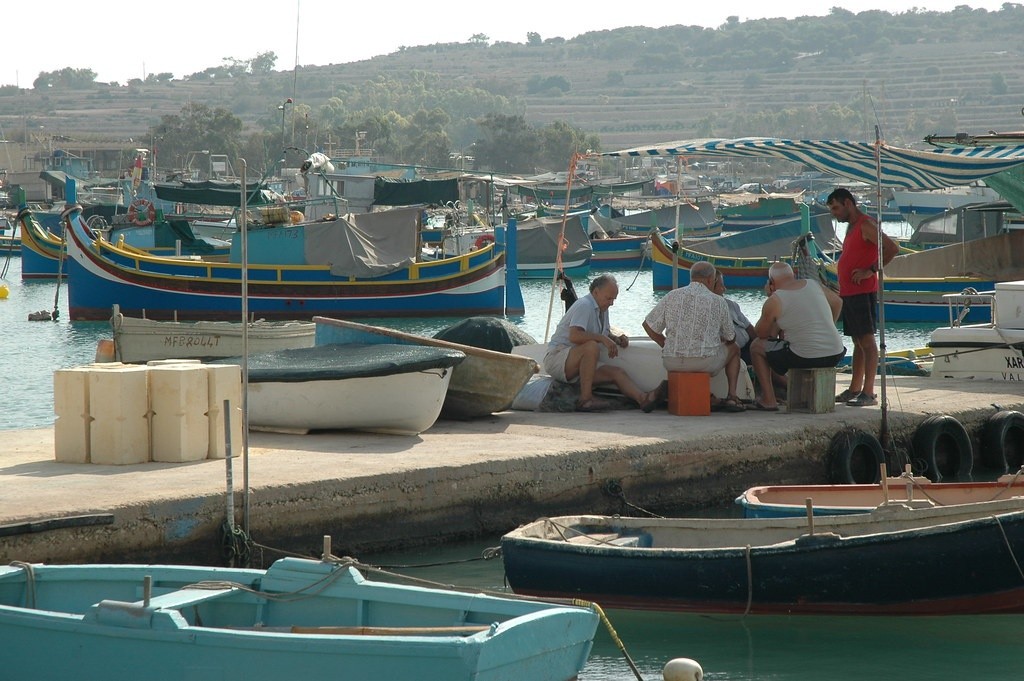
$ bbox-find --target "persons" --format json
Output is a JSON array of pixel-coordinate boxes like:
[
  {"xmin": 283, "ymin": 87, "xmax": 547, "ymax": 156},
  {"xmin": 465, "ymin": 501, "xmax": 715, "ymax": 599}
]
[
  {"xmin": 642, "ymin": 261, "xmax": 749, "ymax": 412},
  {"xmin": 825, "ymin": 187, "xmax": 900, "ymax": 407},
  {"xmin": 543, "ymin": 275, "xmax": 667, "ymax": 413},
  {"xmin": 744, "ymin": 262, "xmax": 847, "ymax": 411},
  {"xmin": 712, "ymin": 268, "xmax": 790, "ymax": 389}
]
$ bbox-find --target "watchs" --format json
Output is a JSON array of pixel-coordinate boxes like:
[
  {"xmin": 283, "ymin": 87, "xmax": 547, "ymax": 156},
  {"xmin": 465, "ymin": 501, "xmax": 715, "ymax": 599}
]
[{"xmin": 871, "ymin": 265, "xmax": 877, "ymax": 273}]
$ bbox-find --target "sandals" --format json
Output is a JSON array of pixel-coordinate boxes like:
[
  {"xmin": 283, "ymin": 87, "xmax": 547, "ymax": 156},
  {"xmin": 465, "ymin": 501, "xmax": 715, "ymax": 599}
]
[
  {"xmin": 834, "ymin": 390, "xmax": 861, "ymax": 402},
  {"xmin": 846, "ymin": 392, "xmax": 878, "ymax": 406},
  {"xmin": 723, "ymin": 397, "xmax": 744, "ymax": 412},
  {"xmin": 641, "ymin": 379, "xmax": 668, "ymax": 413},
  {"xmin": 577, "ymin": 396, "xmax": 609, "ymax": 412},
  {"xmin": 711, "ymin": 394, "xmax": 725, "ymax": 412}
]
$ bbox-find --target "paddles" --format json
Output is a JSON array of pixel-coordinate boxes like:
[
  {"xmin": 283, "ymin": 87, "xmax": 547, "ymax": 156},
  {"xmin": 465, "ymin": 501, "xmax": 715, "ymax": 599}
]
[{"xmin": 227, "ymin": 625, "xmax": 489, "ymax": 636}]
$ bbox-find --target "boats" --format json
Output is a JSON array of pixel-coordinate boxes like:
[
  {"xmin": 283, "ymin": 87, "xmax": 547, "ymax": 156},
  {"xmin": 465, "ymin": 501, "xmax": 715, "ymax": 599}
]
[
  {"xmin": 838, "ymin": 279, "xmax": 1024, "ymax": 381},
  {"xmin": 310, "ymin": 314, "xmax": 540, "ymax": 419},
  {"xmin": 110, "ymin": 304, "xmax": 315, "ymax": 362},
  {"xmin": 500, "ymin": 478, "xmax": 1024, "ymax": 614},
  {"xmin": 1, "ymin": 553, "xmax": 603, "ymax": 680},
  {"xmin": 65, "ymin": 177, "xmax": 523, "ymax": 326},
  {"xmin": 0, "ymin": 128, "xmax": 725, "ymax": 281},
  {"xmin": 648, "ymin": 185, "xmax": 1024, "ymax": 323},
  {"xmin": 214, "ymin": 343, "xmax": 466, "ymax": 439}
]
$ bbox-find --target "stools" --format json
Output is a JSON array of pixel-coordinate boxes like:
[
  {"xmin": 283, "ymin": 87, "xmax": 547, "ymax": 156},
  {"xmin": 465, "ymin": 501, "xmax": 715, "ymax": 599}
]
[
  {"xmin": 667, "ymin": 371, "xmax": 711, "ymax": 417},
  {"xmin": 786, "ymin": 366, "xmax": 836, "ymax": 412}
]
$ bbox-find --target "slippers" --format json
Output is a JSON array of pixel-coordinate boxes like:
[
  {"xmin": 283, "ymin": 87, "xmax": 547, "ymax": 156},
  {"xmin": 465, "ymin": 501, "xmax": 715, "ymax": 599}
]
[{"xmin": 745, "ymin": 400, "xmax": 779, "ymax": 411}]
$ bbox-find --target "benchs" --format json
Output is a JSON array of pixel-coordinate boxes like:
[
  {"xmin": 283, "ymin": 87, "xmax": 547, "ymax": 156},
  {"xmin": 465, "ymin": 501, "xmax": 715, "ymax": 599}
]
[
  {"xmin": 562, "ymin": 534, "xmax": 652, "ymax": 547},
  {"xmin": 133, "ymin": 583, "xmax": 248, "ymax": 610}
]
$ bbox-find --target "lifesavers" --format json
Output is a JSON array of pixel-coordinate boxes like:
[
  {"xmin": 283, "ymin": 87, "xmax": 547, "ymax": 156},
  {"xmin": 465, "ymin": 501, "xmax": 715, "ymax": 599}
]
[
  {"xmin": 475, "ymin": 235, "xmax": 495, "ymax": 249},
  {"xmin": 982, "ymin": 410, "xmax": 1024, "ymax": 471},
  {"xmin": 129, "ymin": 200, "xmax": 155, "ymax": 227},
  {"xmin": 912, "ymin": 415, "xmax": 974, "ymax": 482},
  {"xmin": 828, "ymin": 429, "xmax": 885, "ymax": 484}
]
